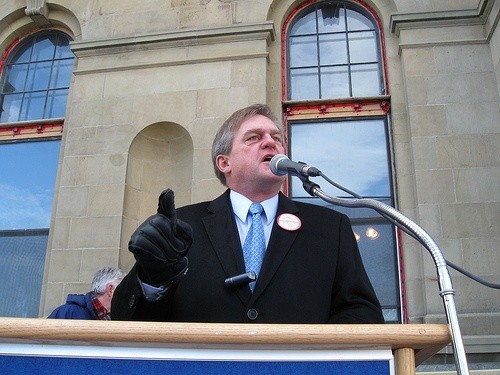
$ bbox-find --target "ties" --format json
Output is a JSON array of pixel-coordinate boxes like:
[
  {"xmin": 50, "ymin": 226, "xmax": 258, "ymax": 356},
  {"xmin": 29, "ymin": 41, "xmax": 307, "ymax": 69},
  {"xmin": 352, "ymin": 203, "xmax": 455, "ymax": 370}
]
[{"xmin": 242, "ymin": 204, "xmax": 266, "ymax": 294}]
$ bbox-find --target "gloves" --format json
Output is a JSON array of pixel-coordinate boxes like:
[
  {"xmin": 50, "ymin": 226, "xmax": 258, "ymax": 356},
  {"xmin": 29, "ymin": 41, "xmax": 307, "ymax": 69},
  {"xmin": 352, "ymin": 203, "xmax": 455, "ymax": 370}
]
[{"xmin": 128, "ymin": 187, "xmax": 194, "ymax": 285}]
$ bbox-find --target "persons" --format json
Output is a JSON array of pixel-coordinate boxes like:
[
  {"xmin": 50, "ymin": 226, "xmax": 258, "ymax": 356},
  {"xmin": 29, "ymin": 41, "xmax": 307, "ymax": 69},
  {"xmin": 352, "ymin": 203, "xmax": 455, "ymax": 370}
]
[
  {"xmin": 47, "ymin": 266, "xmax": 127, "ymax": 320},
  {"xmin": 109, "ymin": 104, "xmax": 385, "ymax": 324}
]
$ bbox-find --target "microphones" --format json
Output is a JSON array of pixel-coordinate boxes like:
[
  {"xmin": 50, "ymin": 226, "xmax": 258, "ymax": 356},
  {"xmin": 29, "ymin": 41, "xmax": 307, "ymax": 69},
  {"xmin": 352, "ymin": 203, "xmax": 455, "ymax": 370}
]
[{"xmin": 269, "ymin": 153, "xmax": 321, "ymax": 178}]
